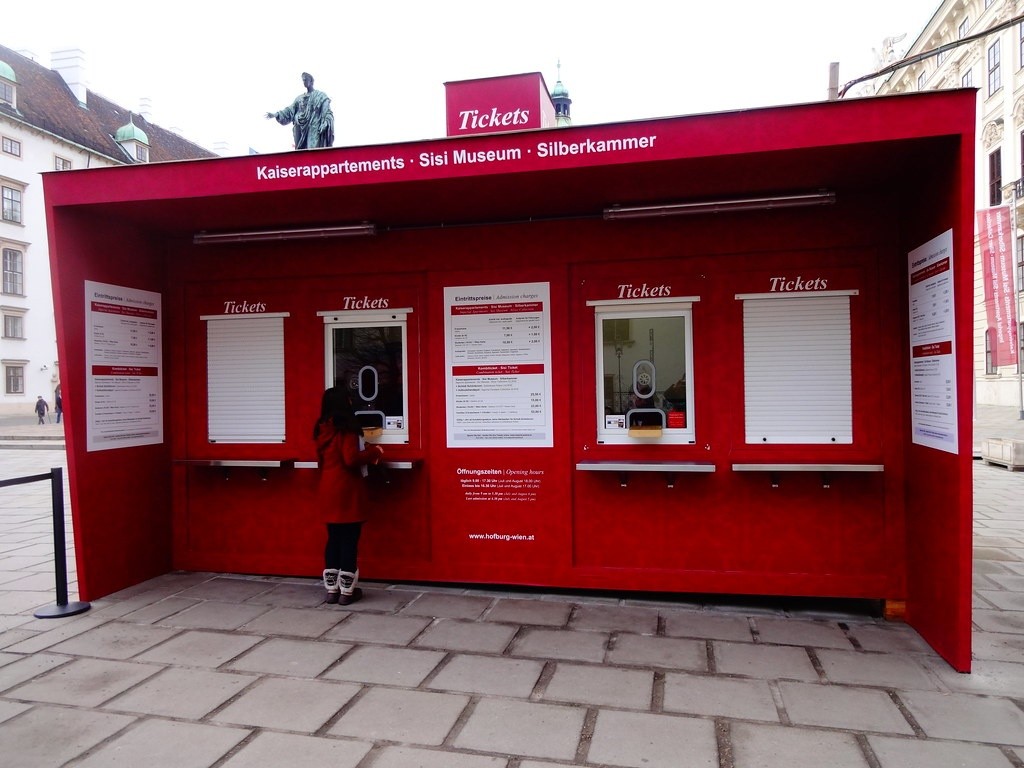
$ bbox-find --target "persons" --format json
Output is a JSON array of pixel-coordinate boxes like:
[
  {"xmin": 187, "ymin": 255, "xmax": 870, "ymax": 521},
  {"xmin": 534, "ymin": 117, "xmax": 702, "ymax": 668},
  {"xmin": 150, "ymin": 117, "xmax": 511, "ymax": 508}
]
[
  {"xmin": 266, "ymin": 73, "xmax": 334, "ymax": 152},
  {"xmin": 312, "ymin": 388, "xmax": 384, "ymax": 606},
  {"xmin": 35, "ymin": 396, "xmax": 50, "ymax": 425},
  {"xmin": 624, "ymin": 390, "xmax": 663, "ymax": 427},
  {"xmin": 664, "ymin": 378, "xmax": 686, "ymax": 412},
  {"xmin": 57, "ymin": 389, "xmax": 63, "ymax": 424}
]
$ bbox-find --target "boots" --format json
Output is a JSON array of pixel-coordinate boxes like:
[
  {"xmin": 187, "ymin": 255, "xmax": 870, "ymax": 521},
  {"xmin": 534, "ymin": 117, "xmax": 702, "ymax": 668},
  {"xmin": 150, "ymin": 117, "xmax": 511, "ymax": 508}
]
[{"xmin": 324, "ymin": 568, "xmax": 365, "ymax": 605}]
